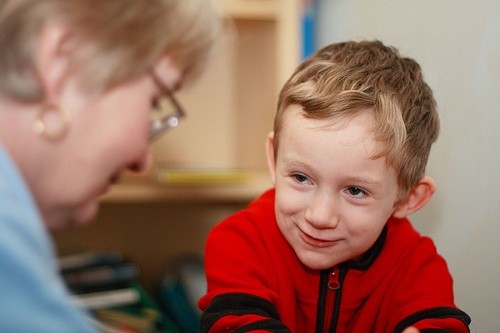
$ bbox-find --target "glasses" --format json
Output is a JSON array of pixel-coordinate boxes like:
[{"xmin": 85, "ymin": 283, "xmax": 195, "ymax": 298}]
[{"xmin": 142, "ymin": 64, "xmax": 187, "ymax": 138}]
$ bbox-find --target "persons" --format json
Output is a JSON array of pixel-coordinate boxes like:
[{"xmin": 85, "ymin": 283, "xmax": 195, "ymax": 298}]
[
  {"xmin": 1, "ymin": 1, "xmax": 239, "ymax": 332},
  {"xmin": 197, "ymin": 40, "xmax": 473, "ymax": 333}
]
